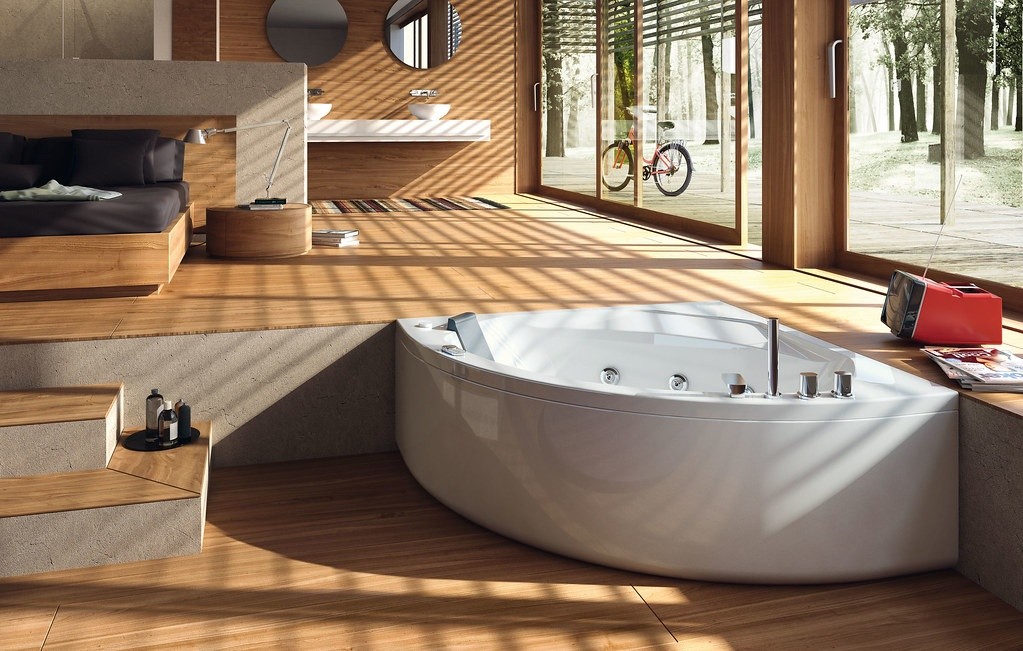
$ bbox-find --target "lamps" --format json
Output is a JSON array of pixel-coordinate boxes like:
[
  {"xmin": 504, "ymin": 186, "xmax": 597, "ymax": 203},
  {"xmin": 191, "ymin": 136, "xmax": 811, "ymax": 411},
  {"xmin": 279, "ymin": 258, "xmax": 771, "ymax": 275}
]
[{"xmin": 182, "ymin": 120, "xmax": 292, "ymax": 208}]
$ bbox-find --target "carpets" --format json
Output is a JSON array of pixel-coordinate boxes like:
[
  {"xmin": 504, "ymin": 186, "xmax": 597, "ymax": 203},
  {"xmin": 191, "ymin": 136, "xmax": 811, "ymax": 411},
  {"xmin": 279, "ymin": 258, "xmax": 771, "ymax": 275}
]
[{"xmin": 310, "ymin": 196, "xmax": 511, "ymax": 215}]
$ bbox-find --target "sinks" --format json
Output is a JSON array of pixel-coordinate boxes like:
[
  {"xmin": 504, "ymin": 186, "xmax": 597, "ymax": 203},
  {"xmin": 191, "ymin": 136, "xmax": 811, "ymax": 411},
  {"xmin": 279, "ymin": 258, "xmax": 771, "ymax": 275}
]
[
  {"xmin": 307, "ymin": 102, "xmax": 332, "ymax": 120},
  {"xmin": 407, "ymin": 103, "xmax": 451, "ymax": 120}
]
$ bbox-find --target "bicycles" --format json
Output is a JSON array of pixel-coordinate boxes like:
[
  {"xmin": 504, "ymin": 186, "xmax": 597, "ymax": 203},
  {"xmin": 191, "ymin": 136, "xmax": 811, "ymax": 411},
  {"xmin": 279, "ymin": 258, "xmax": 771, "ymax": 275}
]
[{"xmin": 603, "ymin": 106, "xmax": 695, "ymax": 197}]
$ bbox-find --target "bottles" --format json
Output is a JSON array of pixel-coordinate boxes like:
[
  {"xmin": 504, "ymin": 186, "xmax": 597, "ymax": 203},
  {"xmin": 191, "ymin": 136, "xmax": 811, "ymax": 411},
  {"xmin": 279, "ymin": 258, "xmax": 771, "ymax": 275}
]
[
  {"xmin": 157, "ymin": 400, "xmax": 178, "ymax": 447},
  {"xmin": 145, "ymin": 388, "xmax": 164, "ymax": 444},
  {"xmin": 174, "ymin": 398, "xmax": 192, "ymax": 440}
]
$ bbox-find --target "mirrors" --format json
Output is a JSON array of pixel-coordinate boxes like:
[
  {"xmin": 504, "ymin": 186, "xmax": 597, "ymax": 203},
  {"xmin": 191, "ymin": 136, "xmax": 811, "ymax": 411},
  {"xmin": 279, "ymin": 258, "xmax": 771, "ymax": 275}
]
[
  {"xmin": 266, "ymin": 0, "xmax": 348, "ymax": 68},
  {"xmin": 384, "ymin": 0, "xmax": 462, "ymax": 70}
]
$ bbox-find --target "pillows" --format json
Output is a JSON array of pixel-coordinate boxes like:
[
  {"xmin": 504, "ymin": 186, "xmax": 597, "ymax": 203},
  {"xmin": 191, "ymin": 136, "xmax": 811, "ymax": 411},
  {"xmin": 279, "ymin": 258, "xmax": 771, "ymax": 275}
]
[
  {"xmin": 0, "ymin": 131, "xmax": 14, "ymax": 160},
  {"xmin": 12, "ymin": 135, "xmax": 26, "ymax": 161},
  {"xmin": 141, "ymin": 129, "xmax": 160, "ymax": 184},
  {"xmin": 28, "ymin": 136, "xmax": 72, "ymax": 186},
  {"xmin": 71, "ymin": 129, "xmax": 150, "ymax": 186},
  {"xmin": 0, "ymin": 161, "xmax": 42, "ymax": 190},
  {"xmin": 154, "ymin": 135, "xmax": 184, "ymax": 182}
]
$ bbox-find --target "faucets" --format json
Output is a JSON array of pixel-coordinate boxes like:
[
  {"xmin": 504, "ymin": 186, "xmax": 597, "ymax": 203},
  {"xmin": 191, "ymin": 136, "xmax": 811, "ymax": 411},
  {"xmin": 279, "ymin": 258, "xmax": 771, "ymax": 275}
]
[
  {"xmin": 421, "ymin": 90, "xmax": 429, "ymax": 99},
  {"xmin": 766, "ymin": 317, "xmax": 783, "ymax": 397}
]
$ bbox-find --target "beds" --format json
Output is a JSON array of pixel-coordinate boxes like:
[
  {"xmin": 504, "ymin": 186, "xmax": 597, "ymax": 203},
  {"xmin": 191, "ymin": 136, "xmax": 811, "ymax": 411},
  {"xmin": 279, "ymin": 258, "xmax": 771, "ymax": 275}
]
[{"xmin": 0, "ymin": 180, "xmax": 194, "ymax": 293}]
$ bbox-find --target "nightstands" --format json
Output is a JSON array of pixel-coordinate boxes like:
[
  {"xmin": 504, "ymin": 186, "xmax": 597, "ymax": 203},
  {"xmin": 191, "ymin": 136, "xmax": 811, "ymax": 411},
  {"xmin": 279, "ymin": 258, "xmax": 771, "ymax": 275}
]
[{"xmin": 206, "ymin": 203, "xmax": 312, "ymax": 260}]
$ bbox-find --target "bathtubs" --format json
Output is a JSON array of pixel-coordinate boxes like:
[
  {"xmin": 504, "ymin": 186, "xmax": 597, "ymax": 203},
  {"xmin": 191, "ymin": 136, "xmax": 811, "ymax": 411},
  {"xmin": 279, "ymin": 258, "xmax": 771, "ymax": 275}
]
[{"xmin": 391, "ymin": 295, "xmax": 964, "ymax": 588}]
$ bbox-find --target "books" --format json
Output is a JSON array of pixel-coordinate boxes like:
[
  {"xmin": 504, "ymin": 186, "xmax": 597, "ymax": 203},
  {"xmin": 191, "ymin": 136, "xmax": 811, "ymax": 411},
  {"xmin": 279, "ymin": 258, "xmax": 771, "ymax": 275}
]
[
  {"xmin": 238, "ymin": 198, "xmax": 286, "ymax": 210},
  {"xmin": 312, "ymin": 230, "xmax": 359, "ymax": 247},
  {"xmin": 920, "ymin": 346, "xmax": 1023, "ymax": 393}
]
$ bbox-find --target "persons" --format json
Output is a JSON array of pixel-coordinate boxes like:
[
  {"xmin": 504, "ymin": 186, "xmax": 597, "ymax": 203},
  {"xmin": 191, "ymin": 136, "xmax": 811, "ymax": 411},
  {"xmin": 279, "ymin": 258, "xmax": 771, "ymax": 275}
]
[{"xmin": 941, "ymin": 349, "xmax": 1017, "ymax": 372}]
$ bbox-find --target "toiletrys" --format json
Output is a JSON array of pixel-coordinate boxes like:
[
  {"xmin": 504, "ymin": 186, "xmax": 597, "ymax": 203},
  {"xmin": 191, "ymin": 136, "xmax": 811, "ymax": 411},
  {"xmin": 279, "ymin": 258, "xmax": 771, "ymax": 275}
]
[{"xmin": 145, "ymin": 388, "xmax": 191, "ymax": 442}]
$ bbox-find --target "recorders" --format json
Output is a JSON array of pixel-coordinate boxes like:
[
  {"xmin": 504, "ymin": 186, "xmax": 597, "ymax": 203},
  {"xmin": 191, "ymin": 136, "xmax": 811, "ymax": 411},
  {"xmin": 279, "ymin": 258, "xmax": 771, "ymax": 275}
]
[{"xmin": 880, "ymin": 175, "xmax": 1002, "ymax": 346}]
